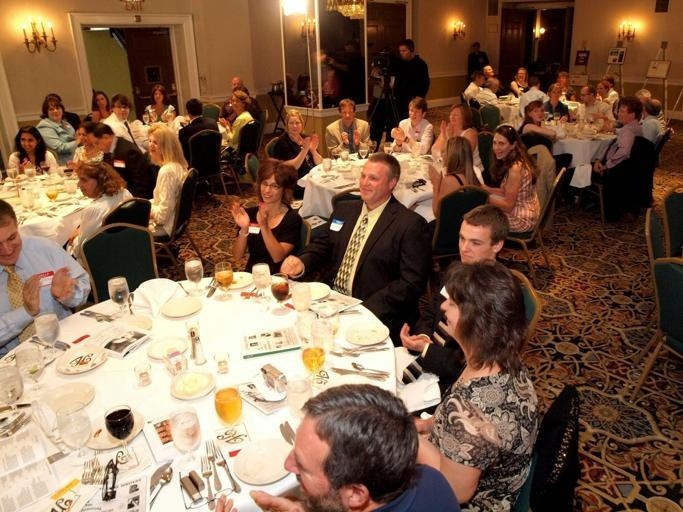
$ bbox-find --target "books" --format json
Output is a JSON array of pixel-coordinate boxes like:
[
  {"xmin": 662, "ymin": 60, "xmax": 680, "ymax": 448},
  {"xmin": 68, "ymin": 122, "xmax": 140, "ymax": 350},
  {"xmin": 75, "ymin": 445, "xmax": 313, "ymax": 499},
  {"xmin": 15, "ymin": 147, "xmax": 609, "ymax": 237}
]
[
  {"xmin": 243, "ymin": 326, "xmax": 301, "ymax": 360},
  {"xmin": 1, "ymin": 423, "xmax": 58, "ymax": 512},
  {"xmin": 308, "ymin": 291, "xmax": 364, "ymax": 319},
  {"xmin": 87, "ymin": 320, "xmax": 151, "ymax": 362}
]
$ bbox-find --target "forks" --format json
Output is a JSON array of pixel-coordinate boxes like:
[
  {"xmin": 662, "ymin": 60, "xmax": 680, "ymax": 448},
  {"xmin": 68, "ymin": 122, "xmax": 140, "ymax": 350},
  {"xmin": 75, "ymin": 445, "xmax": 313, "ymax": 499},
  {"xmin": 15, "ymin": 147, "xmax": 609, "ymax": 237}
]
[
  {"xmin": 81, "ymin": 450, "xmax": 103, "ymax": 490},
  {"xmin": 202, "ymin": 438, "xmax": 240, "ymax": 507}
]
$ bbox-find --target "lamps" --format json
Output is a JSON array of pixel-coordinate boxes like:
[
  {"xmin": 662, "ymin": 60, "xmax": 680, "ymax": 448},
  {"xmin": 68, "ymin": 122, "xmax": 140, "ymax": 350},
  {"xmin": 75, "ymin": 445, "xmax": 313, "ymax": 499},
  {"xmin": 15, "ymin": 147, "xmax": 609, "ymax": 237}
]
[
  {"xmin": 327, "ymin": 0, "xmax": 365, "ymax": 21},
  {"xmin": 536, "ymin": 27, "xmax": 546, "ymax": 40}
]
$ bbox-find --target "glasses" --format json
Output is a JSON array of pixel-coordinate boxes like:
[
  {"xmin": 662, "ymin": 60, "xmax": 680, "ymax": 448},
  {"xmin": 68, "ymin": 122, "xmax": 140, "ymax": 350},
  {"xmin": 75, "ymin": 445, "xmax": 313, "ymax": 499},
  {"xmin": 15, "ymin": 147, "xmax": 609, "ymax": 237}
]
[
  {"xmin": 261, "ymin": 181, "xmax": 281, "ymax": 190},
  {"xmin": 102, "ymin": 459, "xmax": 118, "ymax": 502}
]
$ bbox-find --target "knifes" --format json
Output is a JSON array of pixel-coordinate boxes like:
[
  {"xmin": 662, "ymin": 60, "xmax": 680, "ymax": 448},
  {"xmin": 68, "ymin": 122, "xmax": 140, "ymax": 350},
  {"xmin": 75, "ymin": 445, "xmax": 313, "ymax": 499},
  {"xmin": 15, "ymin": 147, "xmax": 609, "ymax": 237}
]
[
  {"xmin": 149, "ymin": 458, "xmax": 172, "ymax": 490},
  {"xmin": 330, "ymin": 367, "xmax": 386, "ymax": 383},
  {"xmin": 278, "ymin": 421, "xmax": 296, "ymax": 446}
]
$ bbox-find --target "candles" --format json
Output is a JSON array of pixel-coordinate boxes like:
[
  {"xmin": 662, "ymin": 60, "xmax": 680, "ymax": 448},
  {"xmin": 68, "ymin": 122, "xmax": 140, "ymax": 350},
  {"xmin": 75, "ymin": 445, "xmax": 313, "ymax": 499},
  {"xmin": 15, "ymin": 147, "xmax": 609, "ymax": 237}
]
[
  {"xmin": 21, "ymin": 17, "xmax": 57, "ymax": 41},
  {"xmin": 450, "ymin": 22, "xmax": 467, "ymax": 32},
  {"xmin": 299, "ymin": 19, "xmax": 316, "ymax": 34},
  {"xmin": 616, "ymin": 25, "xmax": 636, "ymax": 36}
]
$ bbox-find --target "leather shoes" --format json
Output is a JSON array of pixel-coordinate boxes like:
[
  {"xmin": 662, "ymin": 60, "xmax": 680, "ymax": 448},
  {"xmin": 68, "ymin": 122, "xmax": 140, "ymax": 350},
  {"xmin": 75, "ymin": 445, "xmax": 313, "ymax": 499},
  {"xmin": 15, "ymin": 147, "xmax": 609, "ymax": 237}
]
[{"xmin": 333, "ymin": 213, "xmax": 368, "ymax": 296}]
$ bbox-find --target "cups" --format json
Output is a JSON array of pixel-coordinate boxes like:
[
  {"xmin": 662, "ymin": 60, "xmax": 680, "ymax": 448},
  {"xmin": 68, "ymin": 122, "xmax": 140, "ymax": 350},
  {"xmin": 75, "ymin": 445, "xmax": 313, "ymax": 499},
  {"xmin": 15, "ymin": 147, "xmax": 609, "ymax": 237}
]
[
  {"xmin": 134, "ymin": 361, "xmax": 153, "ymax": 387},
  {"xmin": 211, "ymin": 352, "xmax": 229, "ymax": 375},
  {"xmin": 185, "ymin": 319, "xmax": 198, "ymax": 334}
]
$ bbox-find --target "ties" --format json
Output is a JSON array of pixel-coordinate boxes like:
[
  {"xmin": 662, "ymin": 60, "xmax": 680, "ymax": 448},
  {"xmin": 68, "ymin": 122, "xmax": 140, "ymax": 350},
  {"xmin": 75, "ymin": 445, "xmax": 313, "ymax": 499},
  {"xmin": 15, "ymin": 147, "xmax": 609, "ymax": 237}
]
[
  {"xmin": 3, "ymin": 264, "xmax": 36, "ymax": 344},
  {"xmin": 401, "ymin": 319, "xmax": 447, "ymax": 385}
]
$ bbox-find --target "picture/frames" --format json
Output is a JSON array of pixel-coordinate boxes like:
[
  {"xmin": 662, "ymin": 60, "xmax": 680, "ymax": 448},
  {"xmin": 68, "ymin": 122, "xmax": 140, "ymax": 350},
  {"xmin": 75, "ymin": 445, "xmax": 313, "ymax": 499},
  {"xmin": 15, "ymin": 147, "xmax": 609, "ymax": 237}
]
[
  {"xmin": 646, "ymin": 60, "xmax": 671, "ymax": 79},
  {"xmin": 576, "ymin": 50, "xmax": 590, "ymax": 66},
  {"xmin": 607, "ymin": 47, "xmax": 626, "ymax": 65},
  {"xmin": 144, "ymin": 64, "xmax": 164, "ymax": 84},
  {"xmin": 654, "ymin": 0, "xmax": 671, "ymax": 15}
]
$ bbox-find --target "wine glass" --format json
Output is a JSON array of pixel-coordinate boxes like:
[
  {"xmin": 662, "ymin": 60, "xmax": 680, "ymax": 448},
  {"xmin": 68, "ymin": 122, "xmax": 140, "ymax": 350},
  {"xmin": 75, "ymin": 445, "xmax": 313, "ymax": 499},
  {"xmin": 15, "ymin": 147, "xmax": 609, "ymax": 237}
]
[
  {"xmin": 542, "ymin": 111, "xmax": 603, "ymax": 135},
  {"xmin": 6, "ymin": 160, "xmax": 78, "ymax": 211},
  {"xmin": 303, "ymin": 305, "xmax": 339, "ymax": 389},
  {"xmin": 141, "ymin": 110, "xmax": 173, "ymax": 129},
  {"xmin": 322, "ymin": 140, "xmax": 393, "ymax": 179},
  {"xmin": 184, "ymin": 258, "xmax": 311, "ymax": 330},
  {"xmin": 214, "ymin": 383, "xmax": 243, "ymax": 441},
  {"xmin": 108, "ymin": 276, "xmax": 131, "ymax": 318},
  {"xmin": 0, "ymin": 311, "xmax": 61, "ymax": 416},
  {"xmin": 55, "ymin": 401, "xmax": 94, "ymax": 467},
  {"xmin": 104, "ymin": 405, "xmax": 139, "ymax": 466},
  {"xmin": 168, "ymin": 406, "xmax": 200, "ymax": 470}
]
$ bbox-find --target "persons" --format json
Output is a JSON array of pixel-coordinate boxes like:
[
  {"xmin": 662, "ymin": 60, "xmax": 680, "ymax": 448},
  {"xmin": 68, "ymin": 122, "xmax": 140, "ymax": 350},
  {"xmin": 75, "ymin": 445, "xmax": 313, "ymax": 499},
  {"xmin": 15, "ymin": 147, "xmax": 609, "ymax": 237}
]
[
  {"xmin": 392, "ymin": 42, "xmax": 429, "ymax": 123},
  {"xmin": 9, "ymin": 77, "xmax": 261, "ymax": 267},
  {"xmin": 465, "ymin": 63, "xmax": 665, "ymax": 209},
  {"xmin": 412, "ymin": 256, "xmax": 540, "ymax": 512},
  {"xmin": 280, "ymin": 150, "xmax": 433, "ymax": 343},
  {"xmin": 0, "ymin": 201, "xmax": 93, "ymax": 358},
  {"xmin": 400, "ymin": 199, "xmax": 511, "ymax": 352},
  {"xmin": 214, "ymin": 381, "xmax": 464, "ymax": 512},
  {"xmin": 468, "ymin": 42, "xmax": 489, "ymax": 82},
  {"xmin": 232, "ymin": 100, "xmax": 543, "ymax": 279}
]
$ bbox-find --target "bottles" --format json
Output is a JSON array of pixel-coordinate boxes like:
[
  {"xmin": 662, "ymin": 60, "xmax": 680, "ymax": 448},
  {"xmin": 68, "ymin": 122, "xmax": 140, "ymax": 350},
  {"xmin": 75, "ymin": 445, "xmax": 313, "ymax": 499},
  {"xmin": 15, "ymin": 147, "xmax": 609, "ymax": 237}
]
[{"xmin": 189, "ymin": 327, "xmax": 206, "ymax": 364}]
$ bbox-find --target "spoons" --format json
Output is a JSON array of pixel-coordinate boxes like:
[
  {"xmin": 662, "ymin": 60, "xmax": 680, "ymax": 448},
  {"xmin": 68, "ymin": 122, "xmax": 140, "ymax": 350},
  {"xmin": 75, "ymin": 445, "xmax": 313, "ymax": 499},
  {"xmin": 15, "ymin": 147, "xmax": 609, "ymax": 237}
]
[
  {"xmin": 149, "ymin": 467, "xmax": 173, "ymax": 512},
  {"xmin": 350, "ymin": 361, "xmax": 390, "ymax": 376}
]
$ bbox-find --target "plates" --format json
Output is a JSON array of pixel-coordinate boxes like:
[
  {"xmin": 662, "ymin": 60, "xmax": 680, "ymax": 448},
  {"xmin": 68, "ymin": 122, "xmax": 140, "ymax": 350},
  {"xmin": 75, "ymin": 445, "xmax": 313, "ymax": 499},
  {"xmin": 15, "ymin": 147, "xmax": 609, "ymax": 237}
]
[
  {"xmin": 232, "ymin": 437, "xmax": 294, "ymax": 486},
  {"xmin": 347, "ymin": 322, "xmax": 389, "ymax": 345},
  {"xmin": 144, "ymin": 336, "xmax": 188, "ymax": 359},
  {"xmin": 303, "ymin": 281, "xmax": 330, "ymax": 301},
  {"xmin": 227, "ymin": 271, "xmax": 253, "ymax": 290},
  {"xmin": 169, "ymin": 369, "xmax": 214, "ymax": 400},
  {"xmin": 56, "ymin": 344, "xmax": 108, "ymax": 374},
  {"xmin": 85, "ymin": 409, "xmax": 145, "ymax": 449},
  {"xmin": 163, "ymin": 295, "xmax": 201, "ymax": 317},
  {"xmin": 40, "ymin": 381, "xmax": 95, "ymax": 413}
]
